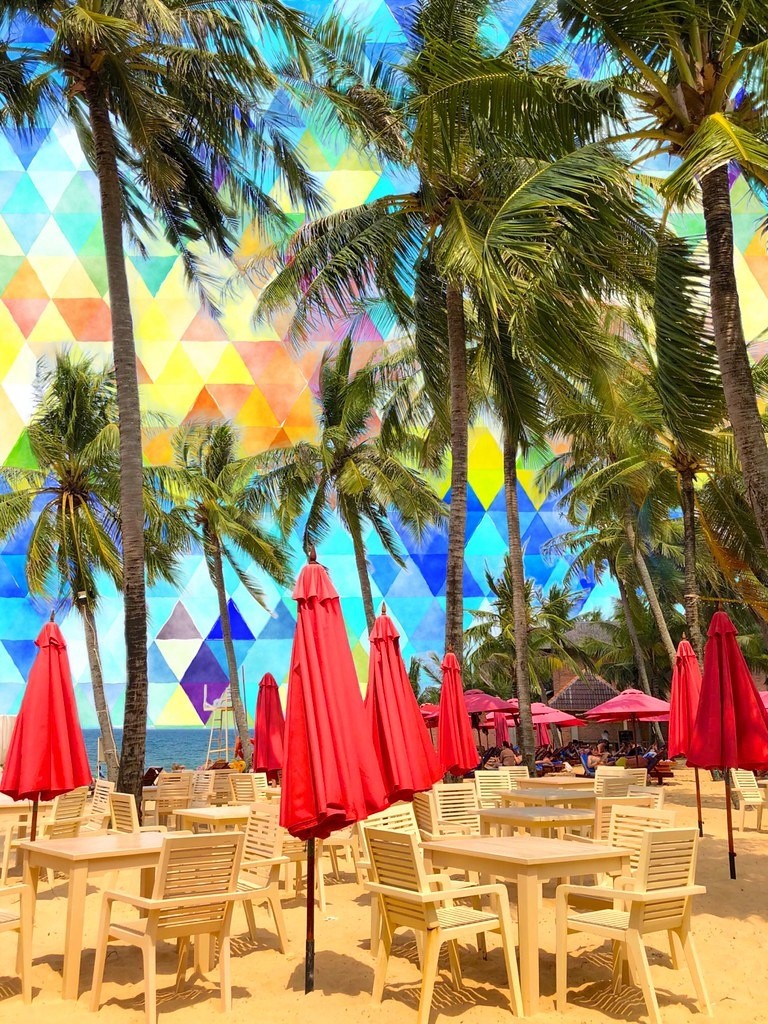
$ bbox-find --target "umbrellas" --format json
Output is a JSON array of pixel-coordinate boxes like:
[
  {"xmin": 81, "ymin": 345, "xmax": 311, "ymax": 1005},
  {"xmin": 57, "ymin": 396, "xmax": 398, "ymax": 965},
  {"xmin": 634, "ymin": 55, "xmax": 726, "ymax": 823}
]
[
  {"xmin": 0, "ymin": 609, "xmax": 94, "ymax": 842},
  {"xmin": 686, "ymin": 598, "xmax": 767, "ymax": 877},
  {"xmin": 363, "ymin": 600, "xmax": 446, "ymax": 804},
  {"xmin": 432, "ymin": 683, "xmax": 672, "ymax": 768},
  {"xmin": 666, "ymin": 630, "xmax": 707, "ymax": 836},
  {"xmin": 436, "ymin": 641, "xmax": 481, "ymax": 784},
  {"xmin": 279, "ymin": 546, "xmax": 390, "ymax": 995},
  {"xmin": 420, "ymin": 702, "xmax": 439, "ymax": 744},
  {"xmin": 253, "ymin": 673, "xmax": 286, "ymax": 786}
]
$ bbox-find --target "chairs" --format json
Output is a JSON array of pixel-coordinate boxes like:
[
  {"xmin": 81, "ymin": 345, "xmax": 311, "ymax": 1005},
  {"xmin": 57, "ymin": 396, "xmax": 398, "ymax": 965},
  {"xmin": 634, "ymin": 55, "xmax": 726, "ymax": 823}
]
[
  {"xmin": 0, "ymin": 743, "xmax": 714, "ymax": 1024},
  {"xmin": 730, "ymin": 767, "xmax": 768, "ymax": 833}
]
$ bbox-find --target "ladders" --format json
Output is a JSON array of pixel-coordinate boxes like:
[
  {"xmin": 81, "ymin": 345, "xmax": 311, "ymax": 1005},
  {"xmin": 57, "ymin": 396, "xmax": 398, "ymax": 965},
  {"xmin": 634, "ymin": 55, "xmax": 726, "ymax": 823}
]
[{"xmin": 203, "ymin": 707, "xmax": 241, "ymax": 770}]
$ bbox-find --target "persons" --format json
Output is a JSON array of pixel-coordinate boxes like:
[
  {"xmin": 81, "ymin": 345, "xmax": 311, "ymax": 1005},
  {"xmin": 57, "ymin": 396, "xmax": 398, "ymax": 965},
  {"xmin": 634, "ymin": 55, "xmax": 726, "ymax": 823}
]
[
  {"xmin": 478, "ymin": 729, "xmax": 670, "ymax": 787},
  {"xmin": 501, "ymin": 739, "xmax": 517, "ymax": 767}
]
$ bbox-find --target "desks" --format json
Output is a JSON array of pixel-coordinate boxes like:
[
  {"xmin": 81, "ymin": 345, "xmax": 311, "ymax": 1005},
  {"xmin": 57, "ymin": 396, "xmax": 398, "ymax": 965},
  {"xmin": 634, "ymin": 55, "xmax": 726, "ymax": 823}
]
[
  {"xmin": 492, "ymin": 787, "xmax": 597, "ymax": 839},
  {"xmin": 513, "ymin": 776, "xmax": 595, "ymax": 837},
  {"xmin": 228, "ymin": 800, "xmax": 279, "ymax": 807},
  {"xmin": 171, "ymin": 806, "xmax": 303, "ymax": 887},
  {"xmin": 0, "ymin": 801, "xmax": 54, "ymax": 876},
  {"xmin": 20, "ymin": 831, "xmax": 209, "ymax": 1007},
  {"xmin": 141, "ymin": 785, "xmax": 173, "ymax": 826},
  {"xmin": 463, "ymin": 806, "xmax": 596, "ymax": 913},
  {"xmin": 417, "ymin": 833, "xmax": 635, "ymax": 1018},
  {"xmin": 257, "ymin": 787, "xmax": 281, "ymax": 799}
]
